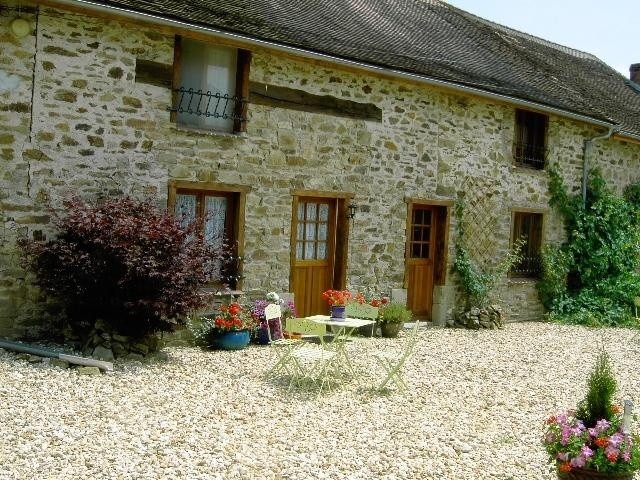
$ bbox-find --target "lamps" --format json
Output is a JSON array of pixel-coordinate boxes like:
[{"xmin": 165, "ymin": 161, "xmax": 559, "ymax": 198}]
[{"xmin": 345, "ymin": 200, "xmax": 357, "ymax": 219}]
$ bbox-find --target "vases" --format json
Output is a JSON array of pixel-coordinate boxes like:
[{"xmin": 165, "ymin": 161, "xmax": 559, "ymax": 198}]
[
  {"xmin": 217, "ymin": 328, "xmax": 251, "ymax": 350},
  {"xmin": 554, "ymin": 461, "xmax": 633, "ymax": 480}
]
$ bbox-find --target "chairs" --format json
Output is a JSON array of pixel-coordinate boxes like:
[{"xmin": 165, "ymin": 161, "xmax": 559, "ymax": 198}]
[{"xmin": 263, "ymin": 300, "xmax": 421, "ymax": 398}]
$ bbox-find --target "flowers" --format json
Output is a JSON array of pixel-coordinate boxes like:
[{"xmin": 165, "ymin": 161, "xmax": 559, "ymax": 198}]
[
  {"xmin": 537, "ymin": 340, "xmax": 640, "ymax": 478},
  {"xmin": 201, "ymin": 285, "xmax": 384, "ymax": 331}
]
